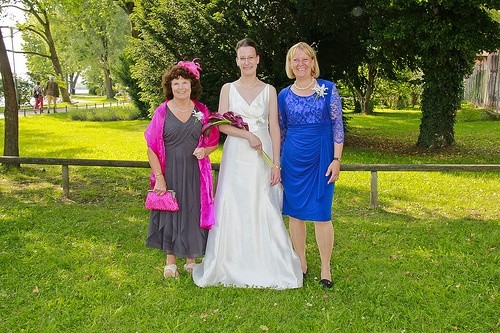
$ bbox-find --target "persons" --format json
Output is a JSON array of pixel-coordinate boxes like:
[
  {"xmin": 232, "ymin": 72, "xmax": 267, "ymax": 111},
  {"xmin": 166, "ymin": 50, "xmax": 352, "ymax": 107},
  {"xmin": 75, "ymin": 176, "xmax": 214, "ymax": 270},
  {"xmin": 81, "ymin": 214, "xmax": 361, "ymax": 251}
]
[
  {"xmin": 34, "ymin": 82, "xmax": 44, "ymax": 113},
  {"xmin": 277, "ymin": 42, "xmax": 344, "ymax": 288},
  {"xmin": 144, "ymin": 61, "xmax": 220, "ymax": 278},
  {"xmin": 43, "ymin": 76, "xmax": 59, "ymax": 114},
  {"xmin": 191, "ymin": 38, "xmax": 303, "ymax": 290}
]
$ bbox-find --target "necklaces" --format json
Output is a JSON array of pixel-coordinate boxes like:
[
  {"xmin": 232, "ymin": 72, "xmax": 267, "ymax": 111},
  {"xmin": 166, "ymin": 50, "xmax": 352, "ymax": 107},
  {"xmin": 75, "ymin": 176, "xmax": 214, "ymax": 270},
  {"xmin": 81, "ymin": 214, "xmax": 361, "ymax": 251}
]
[
  {"xmin": 180, "ymin": 107, "xmax": 191, "ymax": 113},
  {"xmin": 239, "ymin": 77, "xmax": 258, "ymax": 89},
  {"xmin": 294, "ymin": 81, "xmax": 313, "ymax": 90}
]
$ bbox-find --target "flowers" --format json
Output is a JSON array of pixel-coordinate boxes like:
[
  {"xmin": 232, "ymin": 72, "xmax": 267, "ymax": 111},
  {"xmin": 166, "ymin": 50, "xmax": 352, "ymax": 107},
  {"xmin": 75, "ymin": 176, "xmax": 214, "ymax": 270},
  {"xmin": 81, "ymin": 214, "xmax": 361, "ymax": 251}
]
[
  {"xmin": 178, "ymin": 57, "xmax": 203, "ymax": 80},
  {"xmin": 192, "ymin": 110, "xmax": 204, "ymax": 125},
  {"xmin": 201, "ymin": 111, "xmax": 276, "ymax": 168},
  {"xmin": 312, "ymin": 84, "xmax": 328, "ymax": 102}
]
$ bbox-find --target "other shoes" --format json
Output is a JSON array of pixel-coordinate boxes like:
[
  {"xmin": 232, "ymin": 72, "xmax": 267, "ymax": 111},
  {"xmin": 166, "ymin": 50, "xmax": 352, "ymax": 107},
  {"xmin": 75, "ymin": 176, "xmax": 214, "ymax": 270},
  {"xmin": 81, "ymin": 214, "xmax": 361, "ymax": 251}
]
[
  {"xmin": 164, "ymin": 259, "xmax": 180, "ymax": 279},
  {"xmin": 319, "ymin": 279, "xmax": 333, "ymax": 288},
  {"xmin": 183, "ymin": 263, "xmax": 196, "ymax": 274}
]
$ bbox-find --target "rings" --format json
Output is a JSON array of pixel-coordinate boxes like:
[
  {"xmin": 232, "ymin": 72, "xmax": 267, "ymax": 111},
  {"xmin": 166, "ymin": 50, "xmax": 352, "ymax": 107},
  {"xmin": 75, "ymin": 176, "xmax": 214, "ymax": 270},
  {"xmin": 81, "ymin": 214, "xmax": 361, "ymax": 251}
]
[
  {"xmin": 200, "ymin": 156, "xmax": 201, "ymax": 157},
  {"xmin": 337, "ymin": 178, "xmax": 338, "ymax": 179}
]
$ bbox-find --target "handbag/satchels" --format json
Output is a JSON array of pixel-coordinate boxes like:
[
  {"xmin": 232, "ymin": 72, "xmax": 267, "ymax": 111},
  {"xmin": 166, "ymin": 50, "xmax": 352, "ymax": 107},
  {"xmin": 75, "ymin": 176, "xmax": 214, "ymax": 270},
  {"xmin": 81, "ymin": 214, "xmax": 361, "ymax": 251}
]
[{"xmin": 145, "ymin": 190, "xmax": 179, "ymax": 212}]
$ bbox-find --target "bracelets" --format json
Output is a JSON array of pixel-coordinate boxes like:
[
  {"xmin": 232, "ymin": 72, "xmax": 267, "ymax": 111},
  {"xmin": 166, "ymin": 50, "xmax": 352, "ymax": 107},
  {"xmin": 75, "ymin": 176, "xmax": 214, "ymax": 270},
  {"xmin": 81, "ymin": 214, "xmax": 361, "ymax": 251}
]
[
  {"xmin": 274, "ymin": 166, "xmax": 282, "ymax": 171},
  {"xmin": 156, "ymin": 173, "xmax": 162, "ymax": 176}
]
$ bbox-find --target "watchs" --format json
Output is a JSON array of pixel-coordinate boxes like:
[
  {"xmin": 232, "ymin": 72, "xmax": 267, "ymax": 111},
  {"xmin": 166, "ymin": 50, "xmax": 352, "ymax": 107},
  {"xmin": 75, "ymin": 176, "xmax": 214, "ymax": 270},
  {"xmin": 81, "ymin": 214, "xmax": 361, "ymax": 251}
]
[{"xmin": 333, "ymin": 157, "xmax": 342, "ymax": 161}]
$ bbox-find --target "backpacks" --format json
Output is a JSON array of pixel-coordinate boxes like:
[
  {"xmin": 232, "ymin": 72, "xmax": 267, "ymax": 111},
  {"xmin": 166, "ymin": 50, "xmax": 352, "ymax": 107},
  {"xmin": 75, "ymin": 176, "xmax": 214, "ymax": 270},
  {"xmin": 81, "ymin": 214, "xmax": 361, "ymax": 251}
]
[{"xmin": 34, "ymin": 87, "xmax": 40, "ymax": 98}]
